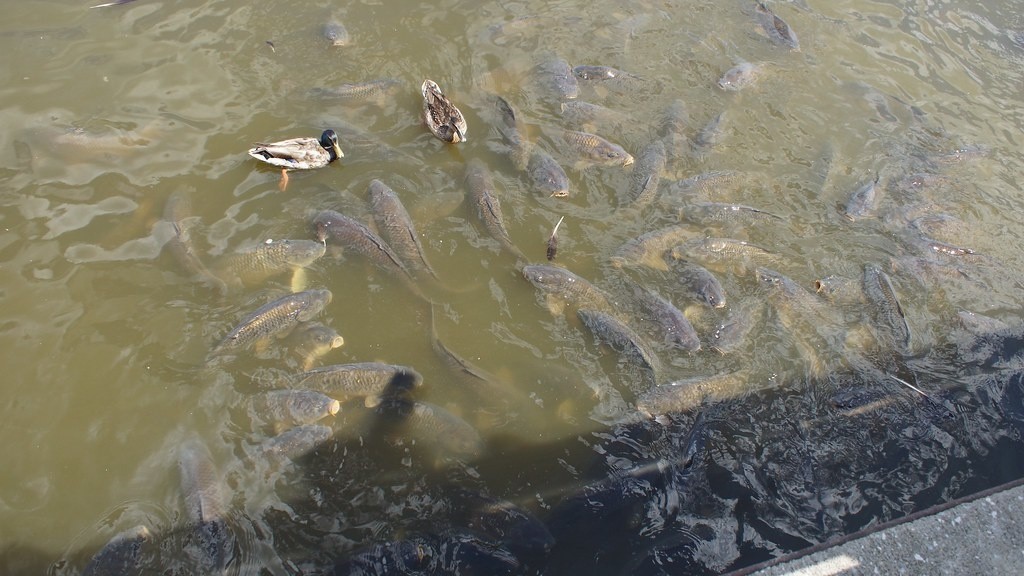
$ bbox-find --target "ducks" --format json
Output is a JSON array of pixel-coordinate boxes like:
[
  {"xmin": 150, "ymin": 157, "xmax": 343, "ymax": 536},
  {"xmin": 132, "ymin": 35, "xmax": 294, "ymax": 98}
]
[
  {"xmin": 248, "ymin": 129, "xmax": 344, "ymax": 192},
  {"xmin": 422, "ymin": 79, "xmax": 468, "ymax": 144}
]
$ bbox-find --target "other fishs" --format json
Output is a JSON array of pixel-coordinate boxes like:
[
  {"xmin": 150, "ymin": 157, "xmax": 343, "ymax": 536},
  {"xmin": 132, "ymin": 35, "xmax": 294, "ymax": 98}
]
[{"xmin": 48, "ymin": 0, "xmax": 1024, "ymax": 559}]
